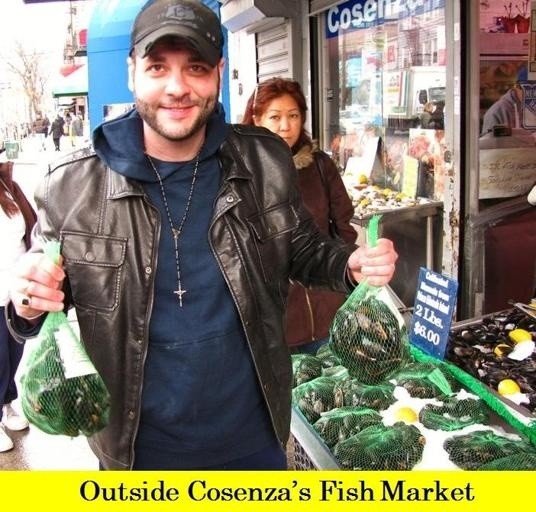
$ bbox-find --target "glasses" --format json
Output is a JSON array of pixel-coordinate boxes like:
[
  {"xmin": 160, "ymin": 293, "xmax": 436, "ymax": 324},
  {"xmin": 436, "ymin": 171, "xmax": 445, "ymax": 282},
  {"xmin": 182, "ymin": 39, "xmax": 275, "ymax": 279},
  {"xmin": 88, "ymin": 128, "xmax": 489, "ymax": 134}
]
[{"xmin": 253, "ymin": 75, "xmax": 297, "ymax": 114}]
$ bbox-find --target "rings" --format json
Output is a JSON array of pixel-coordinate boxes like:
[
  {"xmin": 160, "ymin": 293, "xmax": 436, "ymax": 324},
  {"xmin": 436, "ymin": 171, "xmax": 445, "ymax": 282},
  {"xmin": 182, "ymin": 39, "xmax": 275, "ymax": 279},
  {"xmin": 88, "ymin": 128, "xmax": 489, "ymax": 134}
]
[{"xmin": 20, "ymin": 295, "xmax": 33, "ymax": 310}]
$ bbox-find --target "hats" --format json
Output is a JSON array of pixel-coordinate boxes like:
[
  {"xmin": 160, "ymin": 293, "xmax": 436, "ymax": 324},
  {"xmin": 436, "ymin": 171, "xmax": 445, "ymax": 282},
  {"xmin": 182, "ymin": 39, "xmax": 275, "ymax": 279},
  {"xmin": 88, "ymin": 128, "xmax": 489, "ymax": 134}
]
[{"xmin": 128, "ymin": 0, "xmax": 225, "ymax": 68}]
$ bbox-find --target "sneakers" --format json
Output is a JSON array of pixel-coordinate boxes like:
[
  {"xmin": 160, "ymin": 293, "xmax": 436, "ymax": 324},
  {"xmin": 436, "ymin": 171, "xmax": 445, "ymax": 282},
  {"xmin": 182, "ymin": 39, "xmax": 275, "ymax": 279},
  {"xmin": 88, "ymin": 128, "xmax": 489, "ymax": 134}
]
[
  {"xmin": 0, "ymin": 403, "xmax": 29, "ymax": 431},
  {"xmin": 0, "ymin": 421, "xmax": 14, "ymax": 453}
]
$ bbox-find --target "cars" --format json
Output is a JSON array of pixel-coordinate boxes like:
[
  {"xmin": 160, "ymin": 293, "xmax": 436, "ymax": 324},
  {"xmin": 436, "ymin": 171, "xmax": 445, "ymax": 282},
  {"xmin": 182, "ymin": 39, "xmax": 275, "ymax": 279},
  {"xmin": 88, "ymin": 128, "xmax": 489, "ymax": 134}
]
[
  {"xmin": 337, "ymin": 103, "xmax": 367, "ymax": 132},
  {"xmin": 0, "ymin": 112, "xmax": 53, "ymax": 153}
]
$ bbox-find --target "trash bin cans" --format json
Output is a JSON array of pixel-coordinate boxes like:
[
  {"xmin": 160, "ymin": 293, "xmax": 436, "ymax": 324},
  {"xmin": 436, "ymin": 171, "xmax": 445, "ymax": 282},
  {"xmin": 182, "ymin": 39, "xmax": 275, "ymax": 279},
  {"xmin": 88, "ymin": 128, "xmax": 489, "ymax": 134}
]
[{"xmin": 5, "ymin": 143, "xmax": 18, "ymax": 159}]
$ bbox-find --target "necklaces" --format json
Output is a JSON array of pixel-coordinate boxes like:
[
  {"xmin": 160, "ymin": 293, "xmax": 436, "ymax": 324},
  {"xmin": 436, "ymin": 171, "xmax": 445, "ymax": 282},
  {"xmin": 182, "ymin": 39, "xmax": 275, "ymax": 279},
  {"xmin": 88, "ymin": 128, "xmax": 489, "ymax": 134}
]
[{"xmin": 138, "ymin": 135, "xmax": 205, "ymax": 310}]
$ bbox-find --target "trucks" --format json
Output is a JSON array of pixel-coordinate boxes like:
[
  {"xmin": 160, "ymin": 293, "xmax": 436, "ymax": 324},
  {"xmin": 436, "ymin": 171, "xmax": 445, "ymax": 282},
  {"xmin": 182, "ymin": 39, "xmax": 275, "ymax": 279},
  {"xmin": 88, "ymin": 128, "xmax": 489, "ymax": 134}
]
[{"xmin": 367, "ymin": 65, "xmax": 447, "ymax": 133}]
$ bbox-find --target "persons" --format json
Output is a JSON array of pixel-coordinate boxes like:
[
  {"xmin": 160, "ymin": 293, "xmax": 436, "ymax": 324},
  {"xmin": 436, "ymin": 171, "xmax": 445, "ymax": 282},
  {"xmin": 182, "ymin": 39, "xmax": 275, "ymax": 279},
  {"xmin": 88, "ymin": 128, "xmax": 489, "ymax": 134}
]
[
  {"xmin": 1, "ymin": 161, "xmax": 40, "ymax": 455},
  {"xmin": 49, "ymin": 117, "xmax": 64, "ymax": 152},
  {"xmin": 480, "ymin": 64, "xmax": 529, "ymax": 136},
  {"xmin": 239, "ymin": 78, "xmax": 359, "ymax": 364},
  {"xmin": 3, "ymin": 0, "xmax": 400, "ymax": 472}
]
